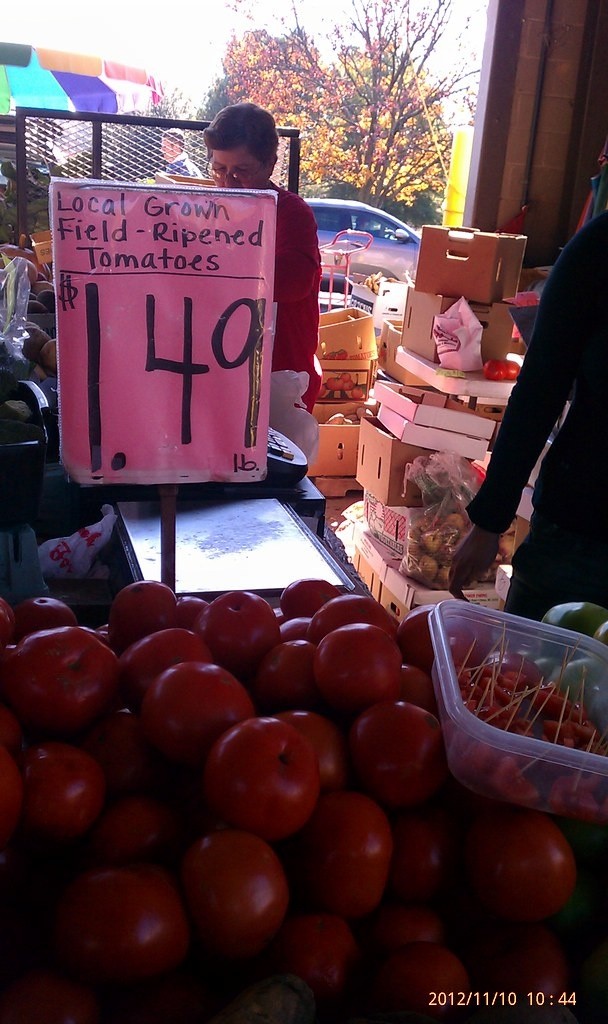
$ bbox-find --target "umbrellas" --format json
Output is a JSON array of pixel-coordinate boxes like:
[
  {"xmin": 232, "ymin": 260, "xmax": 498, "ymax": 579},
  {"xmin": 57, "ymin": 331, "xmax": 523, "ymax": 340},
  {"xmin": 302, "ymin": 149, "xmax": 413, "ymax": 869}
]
[{"xmin": 0, "ymin": 41, "xmax": 165, "ymax": 114}]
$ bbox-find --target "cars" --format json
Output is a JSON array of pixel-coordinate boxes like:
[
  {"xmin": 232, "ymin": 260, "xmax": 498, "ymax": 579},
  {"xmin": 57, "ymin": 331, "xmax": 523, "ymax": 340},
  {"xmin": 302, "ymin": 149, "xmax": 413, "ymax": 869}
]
[{"xmin": 302, "ymin": 197, "xmax": 421, "ymax": 295}]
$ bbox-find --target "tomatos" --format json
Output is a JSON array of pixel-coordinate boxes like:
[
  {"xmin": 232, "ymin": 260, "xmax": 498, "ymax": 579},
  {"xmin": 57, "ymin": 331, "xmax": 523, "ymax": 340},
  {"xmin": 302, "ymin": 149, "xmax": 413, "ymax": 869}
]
[
  {"xmin": 484, "ymin": 359, "xmax": 508, "ymax": 380},
  {"xmin": 505, "ymin": 361, "xmax": 520, "ymax": 380},
  {"xmin": 0, "ymin": 579, "xmax": 577, "ymax": 1024}
]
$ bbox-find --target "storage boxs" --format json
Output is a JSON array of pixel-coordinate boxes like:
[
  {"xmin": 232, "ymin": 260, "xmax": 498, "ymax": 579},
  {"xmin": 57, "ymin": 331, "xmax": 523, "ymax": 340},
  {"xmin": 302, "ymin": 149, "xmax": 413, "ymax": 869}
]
[
  {"xmin": 25, "ymin": 313, "xmax": 56, "ymax": 340},
  {"xmin": 306, "ymin": 225, "xmax": 570, "ymax": 623},
  {"xmin": 30, "ymin": 231, "xmax": 53, "ymax": 265},
  {"xmin": 157, "ymin": 172, "xmax": 217, "ymax": 188},
  {"xmin": 0, "ymin": 381, "xmax": 51, "ymax": 523},
  {"xmin": 428, "ymin": 599, "xmax": 608, "ymax": 822}
]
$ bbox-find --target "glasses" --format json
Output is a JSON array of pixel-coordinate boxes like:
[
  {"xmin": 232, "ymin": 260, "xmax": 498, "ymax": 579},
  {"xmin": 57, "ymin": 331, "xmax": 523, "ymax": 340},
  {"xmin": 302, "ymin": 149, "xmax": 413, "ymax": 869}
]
[{"xmin": 205, "ymin": 157, "xmax": 269, "ymax": 182}]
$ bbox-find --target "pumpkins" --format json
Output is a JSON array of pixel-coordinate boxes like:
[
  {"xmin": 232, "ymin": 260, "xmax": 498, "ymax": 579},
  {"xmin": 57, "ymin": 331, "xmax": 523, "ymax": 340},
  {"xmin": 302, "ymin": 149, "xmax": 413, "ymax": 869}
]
[{"xmin": 0, "ymin": 234, "xmax": 50, "ymax": 281}]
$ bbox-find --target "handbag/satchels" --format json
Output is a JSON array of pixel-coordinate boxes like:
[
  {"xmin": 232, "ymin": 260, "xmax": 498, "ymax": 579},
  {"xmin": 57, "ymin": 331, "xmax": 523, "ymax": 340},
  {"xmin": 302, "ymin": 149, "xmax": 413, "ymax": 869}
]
[
  {"xmin": 32, "ymin": 504, "xmax": 119, "ymax": 584},
  {"xmin": 433, "ymin": 297, "xmax": 484, "ymax": 371}
]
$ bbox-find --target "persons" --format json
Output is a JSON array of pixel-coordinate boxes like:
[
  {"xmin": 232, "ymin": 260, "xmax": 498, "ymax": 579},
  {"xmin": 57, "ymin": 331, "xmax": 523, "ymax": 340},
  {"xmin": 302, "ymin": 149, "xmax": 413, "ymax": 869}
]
[
  {"xmin": 204, "ymin": 103, "xmax": 323, "ymax": 414},
  {"xmin": 447, "ymin": 209, "xmax": 608, "ymax": 622},
  {"xmin": 162, "ymin": 128, "xmax": 204, "ymax": 178},
  {"xmin": 354, "ymin": 216, "xmax": 394, "ymax": 238}
]
